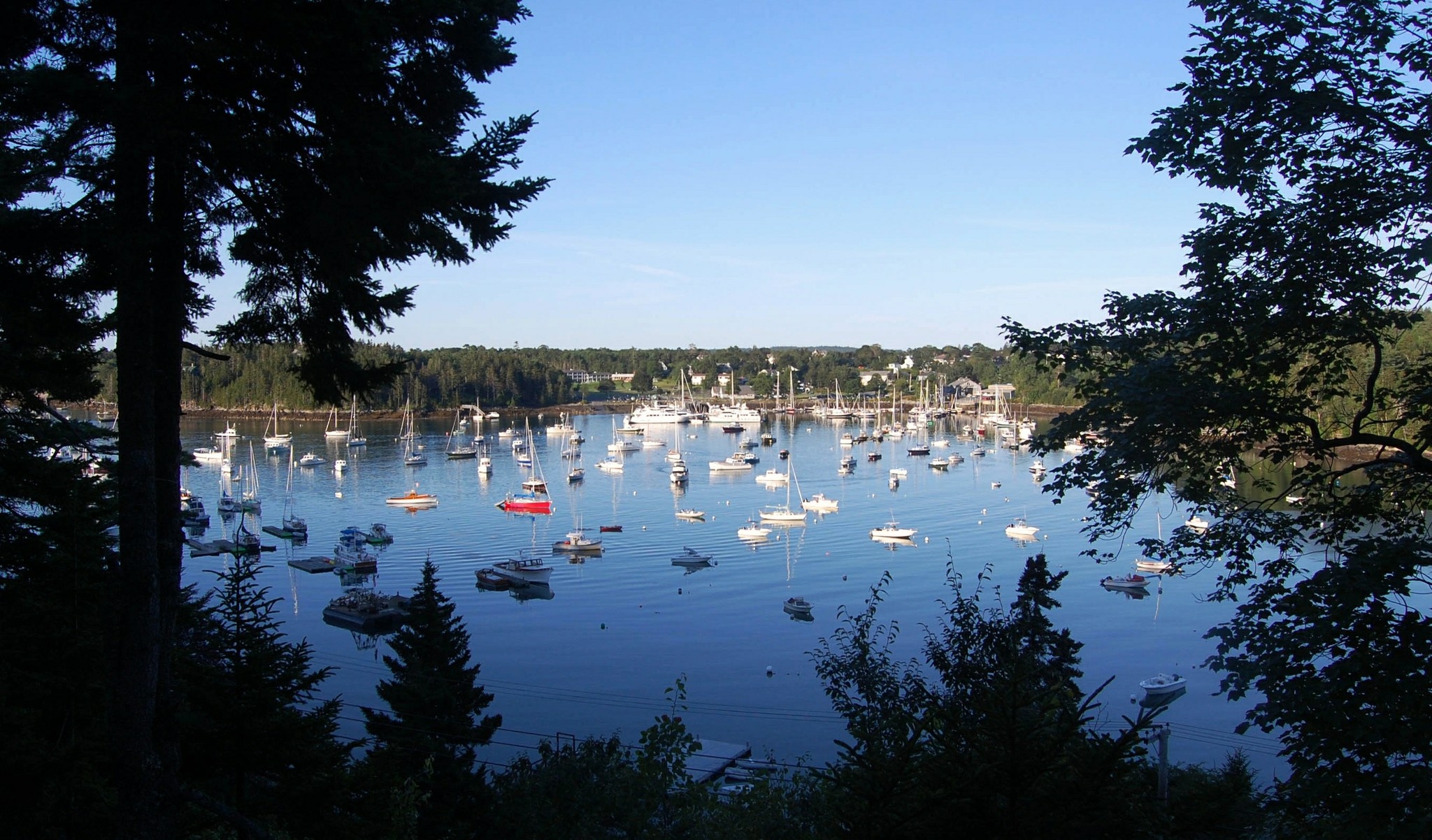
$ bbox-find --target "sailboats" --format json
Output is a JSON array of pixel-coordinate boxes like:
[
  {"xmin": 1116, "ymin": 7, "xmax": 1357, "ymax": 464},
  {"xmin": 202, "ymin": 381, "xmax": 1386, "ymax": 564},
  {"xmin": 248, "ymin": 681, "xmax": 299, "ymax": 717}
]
[
  {"xmin": 1136, "ymin": 568, "xmax": 1182, "ymax": 620},
  {"xmin": 522, "ymin": 430, "xmax": 548, "ymax": 493},
  {"xmin": 759, "ymin": 452, "xmax": 807, "ymax": 520},
  {"xmin": 194, "ymin": 364, "xmax": 1038, "ymax": 515},
  {"xmin": 232, "ymin": 464, "xmax": 261, "ymax": 548},
  {"xmin": 517, "ymin": 416, "xmax": 534, "ymax": 468},
  {"xmin": 282, "ymin": 445, "xmax": 308, "ymax": 534},
  {"xmin": 759, "ymin": 520, "xmax": 807, "ymax": 581},
  {"xmin": 1134, "ymin": 508, "xmax": 1183, "ymax": 570}
]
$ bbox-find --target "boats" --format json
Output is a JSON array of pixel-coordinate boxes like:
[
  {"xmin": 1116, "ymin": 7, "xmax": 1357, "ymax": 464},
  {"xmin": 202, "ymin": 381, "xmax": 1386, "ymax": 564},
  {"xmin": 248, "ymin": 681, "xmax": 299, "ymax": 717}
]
[
  {"xmin": 366, "ymin": 523, "xmax": 394, "ymax": 544},
  {"xmin": 1188, "ymin": 526, "xmax": 1208, "ymax": 536},
  {"xmin": 971, "ymin": 444, "xmax": 986, "ymax": 455},
  {"xmin": 477, "ymin": 444, "xmax": 493, "ymax": 473},
  {"xmin": 31, "ymin": 411, "xmax": 115, "ymax": 487},
  {"xmin": 1139, "ymin": 673, "xmax": 1188, "ymax": 694},
  {"xmin": 670, "ymin": 546, "xmax": 712, "ymax": 563},
  {"xmin": 802, "ymin": 492, "xmax": 839, "ymax": 513},
  {"xmin": 675, "ymin": 508, "xmax": 706, "ymax": 518},
  {"xmin": 889, "ymin": 476, "xmax": 899, "ymax": 486},
  {"xmin": 551, "ymin": 513, "xmax": 603, "ymax": 557},
  {"xmin": 1103, "ymin": 585, "xmax": 1151, "ymax": 601},
  {"xmin": 756, "ymin": 462, "xmax": 788, "ymax": 485},
  {"xmin": 333, "ymin": 526, "xmax": 379, "ymax": 569},
  {"xmin": 478, "ymin": 472, "xmax": 493, "ymax": 480},
  {"xmin": 783, "ymin": 607, "xmax": 815, "ymax": 624},
  {"xmin": 906, "ymin": 422, "xmax": 930, "ymax": 453},
  {"xmin": 928, "ymin": 457, "xmax": 949, "ymax": 467},
  {"xmin": 889, "ymin": 467, "xmax": 908, "ymax": 476},
  {"xmin": 1032, "ymin": 469, "xmax": 1046, "ymax": 478},
  {"xmin": 839, "ymin": 448, "xmax": 857, "ymax": 465},
  {"xmin": 489, "ymin": 550, "xmax": 554, "ymax": 584},
  {"xmin": 906, "ymin": 452, "xmax": 931, "ymax": 460},
  {"xmin": 669, "ymin": 459, "xmax": 690, "ymax": 483},
  {"xmin": 494, "ymin": 491, "xmax": 553, "ymax": 509},
  {"xmin": 870, "ymin": 506, "xmax": 917, "ymax": 537},
  {"xmin": 1286, "ymin": 496, "xmax": 1306, "ymax": 505},
  {"xmin": 867, "ymin": 452, "xmax": 882, "ymax": 459},
  {"xmin": 947, "ymin": 452, "xmax": 964, "ymax": 461},
  {"xmin": 991, "ymin": 482, "xmax": 1002, "ymax": 487},
  {"xmin": 510, "ymin": 581, "xmax": 555, "ymax": 601},
  {"xmin": 474, "ymin": 568, "xmax": 510, "ymax": 589},
  {"xmin": 1061, "ymin": 430, "xmax": 1110, "ymax": 455},
  {"xmin": 181, "ymin": 497, "xmax": 210, "ymax": 529},
  {"xmin": 386, "ymin": 482, "xmax": 439, "ymax": 509},
  {"xmin": 782, "ymin": 595, "xmax": 815, "ymax": 614},
  {"xmin": 1138, "ymin": 687, "xmax": 1187, "ymax": 709},
  {"xmin": 1100, "ymin": 571, "xmax": 1151, "ymax": 588},
  {"xmin": 1185, "ymin": 514, "xmax": 1209, "ymax": 528},
  {"xmin": 838, "ymin": 468, "xmax": 854, "ymax": 476},
  {"xmin": 1005, "ymin": 509, "xmax": 1040, "ymax": 536},
  {"xmin": 1029, "ymin": 459, "xmax": 1047, "ymax": 471},
  {"xmin": 737, "ymin": 523, "xmax": 772, "ymax": 536},
  {"xmin": 1210, "ymin": 457, "xmax": 1236, "ymax": 489}
]
